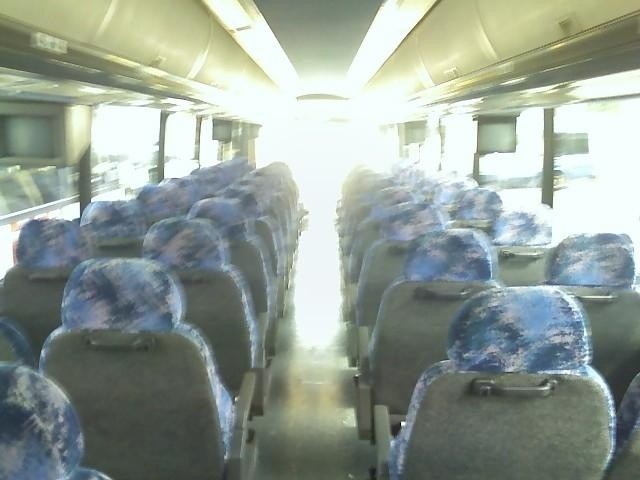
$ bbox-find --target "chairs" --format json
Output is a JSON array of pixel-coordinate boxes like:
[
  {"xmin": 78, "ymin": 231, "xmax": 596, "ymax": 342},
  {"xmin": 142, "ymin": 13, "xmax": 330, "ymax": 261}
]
[
  {"xmin": 0, "ymin": 159, "xmax": 305, "ymax": 479},
  {"xmin": 335, "ymin": 162, "xmax": 640, "ymax": 480}
]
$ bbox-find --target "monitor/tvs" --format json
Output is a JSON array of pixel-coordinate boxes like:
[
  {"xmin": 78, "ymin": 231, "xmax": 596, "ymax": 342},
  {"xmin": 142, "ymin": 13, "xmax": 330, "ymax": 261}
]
[
  {"xmin": 476, "ymin": 117, "xmax": 518, "ymax": 154},
  {"xmin": 1, "ymin": 102, "xmax": 94, "ymax": 170},
  {"xmin": 212, "ymin": 120, "xmax": 263, "ymax": 142}
]
